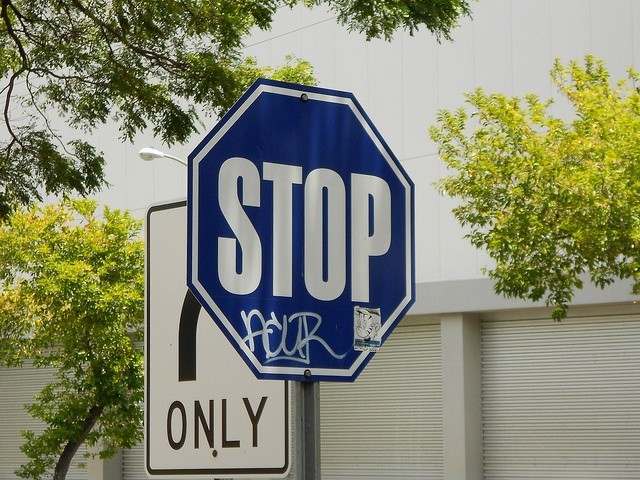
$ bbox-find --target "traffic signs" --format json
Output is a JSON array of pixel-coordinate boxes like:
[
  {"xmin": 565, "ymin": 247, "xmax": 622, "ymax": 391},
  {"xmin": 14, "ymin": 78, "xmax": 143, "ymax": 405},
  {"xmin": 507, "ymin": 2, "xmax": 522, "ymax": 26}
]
[{"xmin": 144, "ymin": 208, "xmax": 292, "ymax": 472}]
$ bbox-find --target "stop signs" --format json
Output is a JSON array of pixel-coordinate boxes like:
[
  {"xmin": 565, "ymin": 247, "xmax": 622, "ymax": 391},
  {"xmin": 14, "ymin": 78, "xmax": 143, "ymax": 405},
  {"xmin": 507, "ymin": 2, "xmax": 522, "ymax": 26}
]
[{"xmin": 186, "ymin": 79, "xmax": 417, "ymax": 383}]
